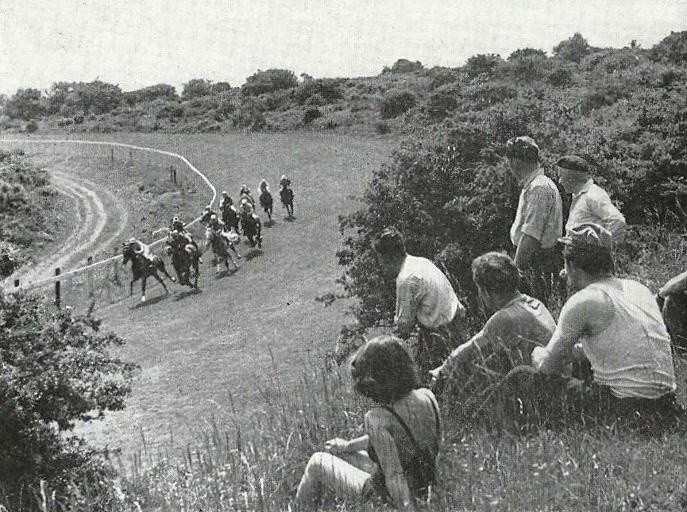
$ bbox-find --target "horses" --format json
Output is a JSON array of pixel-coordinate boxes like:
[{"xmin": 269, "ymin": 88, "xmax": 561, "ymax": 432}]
[
  {"xmin": 199, "ymin": 214, "xmax": 211, "ymax": 225},
  {"xmin": 205, "ymin": 224, "xmax": 242, "ymax": 277},
  {"xmin": 279, "ymin": 188, "xmax": 293, "ymax": 216},
  {"xmin": 240, "ymin": 212, "xmax": 262, "ymax": 249},
  {"xmin": 122, "ymin": 242, "xmax": 177, "ymax": 302},
  {"xmin": 238, "ymin": 193, "xmax": 256, "ymax": 211},
  {"xmin": 259, "ymin": 191, "xmax": 272, "ymax": 223},
  {"xmin": 222, "ymin": 202, "xmax": 241, "ymax": 236},
  {"xmin": 164, "ymin": 236, "xmax": 198, "ymax": 288}
]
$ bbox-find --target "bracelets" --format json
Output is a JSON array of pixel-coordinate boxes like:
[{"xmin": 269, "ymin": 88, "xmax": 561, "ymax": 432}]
[{"xmin": 656, "ymin": 290, "xmax": 665, "ymax": 302}]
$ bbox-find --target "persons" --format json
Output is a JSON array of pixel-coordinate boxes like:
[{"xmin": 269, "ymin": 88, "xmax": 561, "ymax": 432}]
[
  {"xmin": 426, "ymin": 249, "xmax": 572, "ymax": 395},
  {"xmin": 451, "ymin": 231, "xmax": 681, "ymax": 437},
  {"xmin": 651, "ymin": 269, "xmax": 686, "ymax": 352},
  {"xmin": 503, "ymin": 135, "xmax": 565, "ymax": 276},
  {"xmin": 552, "ymin": 154, "xmax": 628, "ymax": 254},
  {"xmin": 370, "ymin": 224, "xmax": 470, "ymax": 353},
  {"xmin": 123, "ymin": 171, "xmax": 296, "ymax": 265},
  {"xmin": 286, "ymin": 332, "xmax": 446, "ymax": 511}
]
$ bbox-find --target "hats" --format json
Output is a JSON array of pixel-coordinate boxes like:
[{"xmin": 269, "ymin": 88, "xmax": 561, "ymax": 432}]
[
  {"xmin": 558, "ymin": 223, "xmax": 613, "ymax": 251},
  {"xmin": 555, "ymin": 156, "xmax": 590, "ymax": 171}
]
